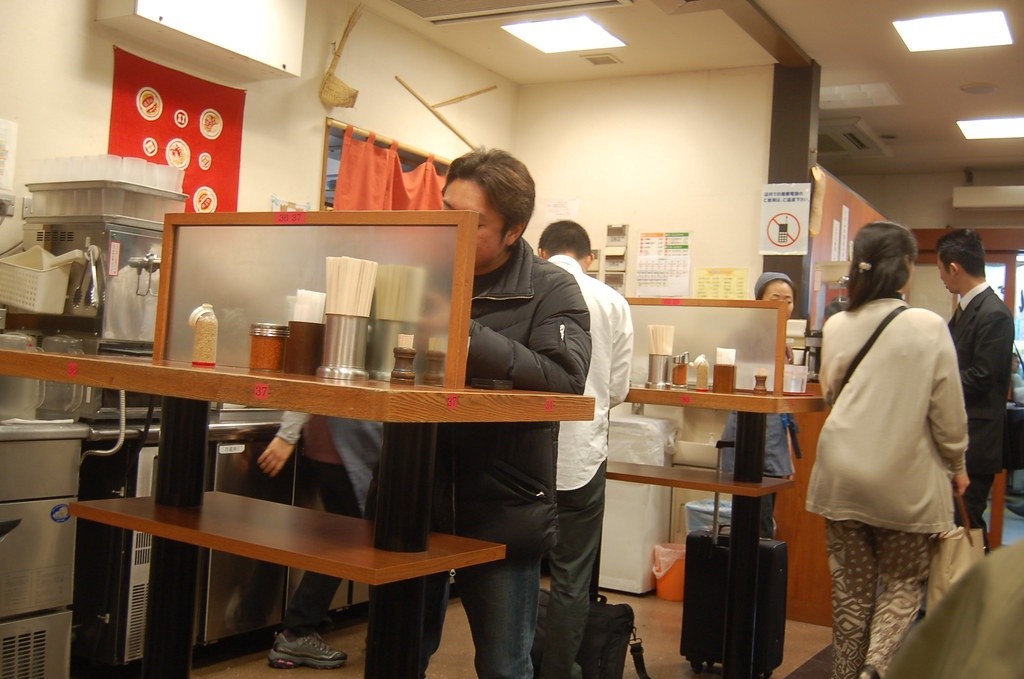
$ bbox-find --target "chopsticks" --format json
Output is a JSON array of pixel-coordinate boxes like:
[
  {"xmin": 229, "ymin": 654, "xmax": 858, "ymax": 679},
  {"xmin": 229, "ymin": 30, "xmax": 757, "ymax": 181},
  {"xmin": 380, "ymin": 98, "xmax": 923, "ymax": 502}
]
[
  {"xmin": 325, "ymin": 256, "xmax": 378, "ymax": 317},
  {"xmin": 648, "ymin": 325, "xmax": 675, "ymax": 355},
  {"xmin": 371, "ymin": 263, "xmax": 425, "ymax": 322}
]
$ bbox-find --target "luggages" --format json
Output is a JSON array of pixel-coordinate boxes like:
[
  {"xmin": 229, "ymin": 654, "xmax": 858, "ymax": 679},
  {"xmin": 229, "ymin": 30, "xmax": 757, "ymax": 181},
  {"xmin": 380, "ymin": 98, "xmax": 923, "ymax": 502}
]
[{"xmin": 679, "ymin": 434, "xmax": 788, "ymax": 677}]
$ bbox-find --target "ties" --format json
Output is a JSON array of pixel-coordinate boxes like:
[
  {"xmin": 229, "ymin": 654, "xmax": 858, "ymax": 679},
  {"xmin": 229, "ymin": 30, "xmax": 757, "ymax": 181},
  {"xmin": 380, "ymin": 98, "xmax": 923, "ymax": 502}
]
[{"xmin": 956, "ymin": 303, "xmax": 963, "ymax": 325}]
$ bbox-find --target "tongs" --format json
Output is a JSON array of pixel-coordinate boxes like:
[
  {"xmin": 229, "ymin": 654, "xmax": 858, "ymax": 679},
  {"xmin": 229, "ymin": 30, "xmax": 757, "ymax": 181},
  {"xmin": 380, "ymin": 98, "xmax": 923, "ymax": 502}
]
[{"xmin": 72, "ymin": 249, "xmax": 96, "ymax": 309}]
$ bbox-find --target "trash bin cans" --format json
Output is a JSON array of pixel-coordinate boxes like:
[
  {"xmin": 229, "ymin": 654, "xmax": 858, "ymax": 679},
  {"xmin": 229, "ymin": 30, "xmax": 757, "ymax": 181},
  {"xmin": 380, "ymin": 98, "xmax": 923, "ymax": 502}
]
[{"xmin": 654, "ymin": 542, "xmax": 685, "ymax": 600}]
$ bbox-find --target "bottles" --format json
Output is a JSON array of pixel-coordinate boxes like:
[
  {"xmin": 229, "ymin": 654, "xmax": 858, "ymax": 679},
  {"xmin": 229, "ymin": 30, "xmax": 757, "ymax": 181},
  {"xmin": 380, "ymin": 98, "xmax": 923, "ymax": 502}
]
[
  {"xmin": 190, "ymin": 303, "xmax": 219, "ymax": 369},
  {"xmin": 695, "ymin": 354, "xmax": 709, "ymax": 392},
  {"xmin": 672, "ymin": 355, "xmax": 688, "ymax": 388}
]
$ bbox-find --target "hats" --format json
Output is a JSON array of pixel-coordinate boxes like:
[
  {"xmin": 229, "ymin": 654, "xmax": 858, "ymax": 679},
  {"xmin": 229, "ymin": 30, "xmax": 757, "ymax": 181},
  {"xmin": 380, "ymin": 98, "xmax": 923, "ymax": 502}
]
[{"xmin": 754, "ymin": 271, "xmax": 793, "ymax": 301}]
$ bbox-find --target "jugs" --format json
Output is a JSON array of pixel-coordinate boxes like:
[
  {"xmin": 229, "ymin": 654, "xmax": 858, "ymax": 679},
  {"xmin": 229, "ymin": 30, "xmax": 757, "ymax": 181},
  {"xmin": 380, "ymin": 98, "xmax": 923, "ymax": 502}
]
[
  {"xmin": 39, "ymin": 335, "xmax": 86, "ymax": 423},
  {"xmin": 0, "ymin": 332, "xmax": 46, "ymax": 422}
]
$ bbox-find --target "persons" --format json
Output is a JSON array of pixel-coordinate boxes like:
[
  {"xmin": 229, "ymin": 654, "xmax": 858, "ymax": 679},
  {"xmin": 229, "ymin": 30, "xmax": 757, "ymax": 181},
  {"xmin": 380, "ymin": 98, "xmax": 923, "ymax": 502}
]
[
  {"xmin": 257, "ymin": 410, "xmax": 384, "ymax": 669},
  {"xmin": 358, "ymin": 145, "xmax": 590, "ymax": 679},
  {"xmin": 937, "ymin": 227, "xmax": 1019, "ymax": 562},
  {"xmin": 722, "ymin": 271, "xmax": 800, "ymax": 540},
  {"xmin": 805, "ymin": 221, "xmax": 970, "ymax": 678},
  {"xmin": 883, "ymin": 539, "xmax": 1024, "ymax": 679},
  {"xmin": 535, "ymin": 220, "xmax": 634, "ymax": 679}
]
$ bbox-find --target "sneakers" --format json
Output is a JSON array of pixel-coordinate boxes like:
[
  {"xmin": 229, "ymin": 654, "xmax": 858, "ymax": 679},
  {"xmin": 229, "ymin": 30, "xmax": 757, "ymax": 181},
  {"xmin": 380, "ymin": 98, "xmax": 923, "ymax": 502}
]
[{"xmin": 266, "ymin": 628, "xmax": 348, "ymax": 667}]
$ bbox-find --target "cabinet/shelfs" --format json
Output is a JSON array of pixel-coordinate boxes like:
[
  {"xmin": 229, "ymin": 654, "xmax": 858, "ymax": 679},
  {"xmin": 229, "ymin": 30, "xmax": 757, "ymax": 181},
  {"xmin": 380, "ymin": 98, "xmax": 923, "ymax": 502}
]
[
  {"xmin": 605, "ymin": 298, "xmax": 825, "ymax": 679},
  {"xmin": 0, "ymin": 213, "xmax": 595, "ymax": 679},
  {"xmin": 94, "ymin": 0, "xmax": 308, "ymax": 78}
]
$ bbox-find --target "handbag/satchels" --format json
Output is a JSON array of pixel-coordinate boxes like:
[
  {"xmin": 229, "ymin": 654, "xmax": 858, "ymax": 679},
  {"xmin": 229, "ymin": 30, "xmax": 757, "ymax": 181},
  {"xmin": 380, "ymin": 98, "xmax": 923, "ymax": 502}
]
[
  {"xmin": 925, "ymin": 489, "xmax": 983, "ymax": 617},
  {"xmin": 532, "ymin": 584, "xmax": 650, "ymax": 679}
]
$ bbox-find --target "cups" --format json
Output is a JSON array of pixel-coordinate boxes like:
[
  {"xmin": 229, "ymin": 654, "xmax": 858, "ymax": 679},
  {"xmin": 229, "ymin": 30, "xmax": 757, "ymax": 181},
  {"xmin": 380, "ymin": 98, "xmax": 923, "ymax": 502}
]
[
  {"xmin": 368, "ymin": 318, "xmax": 420, "ymax": 379},
  {"xmin": 713, "ymin": 364, "xmax": 737, "ymax": 394},
  {"xmin": 27, "ymin": 155, "xmax": 185, "ymax": 193},
  {"xmin": 645, "ymin": 353, "xmax": 673, "ymax": 390},
  {"xmin": 249, "ymin": 311, "xmax": 371, "ymax": 382}
]
